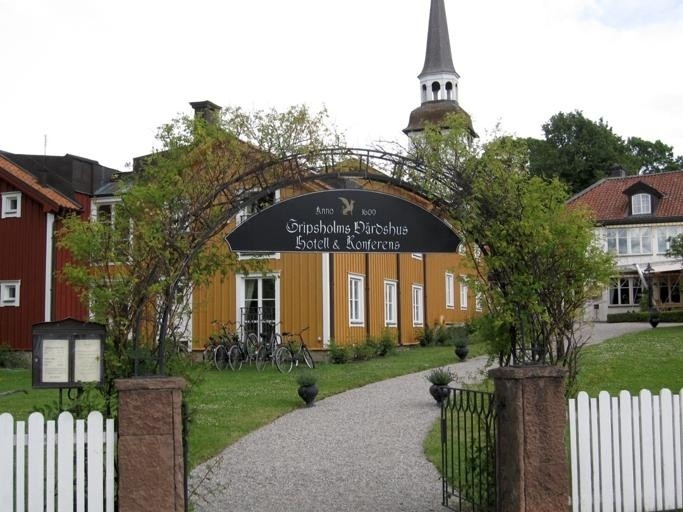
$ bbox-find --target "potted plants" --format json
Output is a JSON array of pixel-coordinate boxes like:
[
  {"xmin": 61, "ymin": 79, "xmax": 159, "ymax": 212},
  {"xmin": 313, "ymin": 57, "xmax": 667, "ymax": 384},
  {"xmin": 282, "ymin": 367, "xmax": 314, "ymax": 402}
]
[
  {"xmin": 453, "ymin": 338, "xmax": 469, "ymax": 360},
  {"xmin": 295, "ymin": 373, "xmax": 318, "ymax": 405},
  {"xmin": 426, "ymin": 369, "xmax": 456, "ymax": 405}
]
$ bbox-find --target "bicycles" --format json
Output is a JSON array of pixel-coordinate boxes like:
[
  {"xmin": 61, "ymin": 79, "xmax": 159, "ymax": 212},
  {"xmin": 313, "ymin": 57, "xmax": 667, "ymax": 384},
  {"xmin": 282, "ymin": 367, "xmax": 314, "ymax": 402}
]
[
  {"xmin": 274, "ymin": 326, "xmax": 316, "ymax": 374},
  {"xmin": 202, "ymin": 318, "xmax": 283, "ymax": 373}
]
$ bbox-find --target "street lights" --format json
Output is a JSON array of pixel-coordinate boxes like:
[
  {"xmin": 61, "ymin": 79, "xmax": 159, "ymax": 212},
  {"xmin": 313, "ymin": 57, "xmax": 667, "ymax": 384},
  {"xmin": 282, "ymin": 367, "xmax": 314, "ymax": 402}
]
[{"xmin": 644, "ymin": 263, "xmax": 654, "ymax": 311}]
